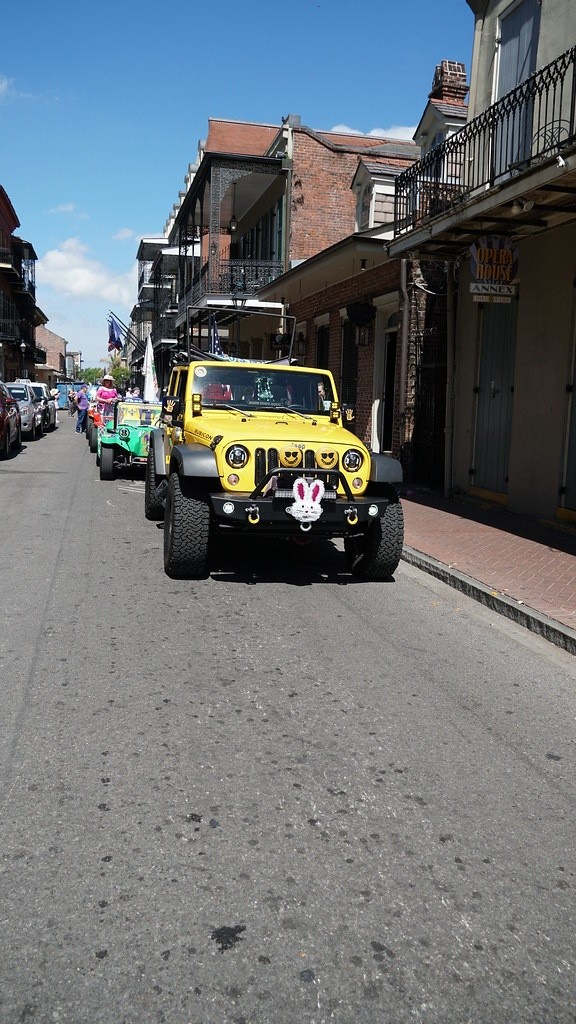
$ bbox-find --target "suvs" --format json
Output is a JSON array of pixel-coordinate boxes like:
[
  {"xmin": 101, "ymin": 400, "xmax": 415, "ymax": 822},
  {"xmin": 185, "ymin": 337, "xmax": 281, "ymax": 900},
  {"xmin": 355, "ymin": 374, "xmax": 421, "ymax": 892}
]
[{"xmin": 143, "ymin": 310, "xmax": 410, "ymax": 581}]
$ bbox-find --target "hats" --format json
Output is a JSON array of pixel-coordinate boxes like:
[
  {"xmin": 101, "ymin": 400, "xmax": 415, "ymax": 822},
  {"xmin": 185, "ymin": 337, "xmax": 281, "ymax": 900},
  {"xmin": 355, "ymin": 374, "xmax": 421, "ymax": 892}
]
[
  {"xmin": 81, "ymin": 384, "xmax": 88, "ymax": 389},
  {"xmin": 100, "ymin": 375, "xmax": 115, "ymax": 385}
]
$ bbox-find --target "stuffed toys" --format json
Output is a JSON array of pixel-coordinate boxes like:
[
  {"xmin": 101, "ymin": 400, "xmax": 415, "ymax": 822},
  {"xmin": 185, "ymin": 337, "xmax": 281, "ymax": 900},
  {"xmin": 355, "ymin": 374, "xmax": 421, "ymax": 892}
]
[{"xmin": 291, "ymin": 479, "xmax": 325, "ymax": 522}]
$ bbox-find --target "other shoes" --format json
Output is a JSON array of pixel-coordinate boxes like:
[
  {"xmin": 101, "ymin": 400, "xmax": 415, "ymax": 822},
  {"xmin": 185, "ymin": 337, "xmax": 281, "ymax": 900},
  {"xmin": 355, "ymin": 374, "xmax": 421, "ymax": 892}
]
[
  {"xmin": 76, "ymin": 430, "xmax": 80, "ymax": 434},
  {"xmin": 82, "ymin": 432, "xmax": 86, "ymax": 434}
]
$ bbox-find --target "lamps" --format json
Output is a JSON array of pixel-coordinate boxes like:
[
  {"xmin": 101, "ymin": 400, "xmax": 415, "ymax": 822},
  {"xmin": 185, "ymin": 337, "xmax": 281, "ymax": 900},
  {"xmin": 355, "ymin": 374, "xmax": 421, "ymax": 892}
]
[
  {"xmin": 359, "ymin": 259, "xmax": 367, "ymax": 271},
  {"xmin": 229, "ymin": 183, "xmax": 238, "ymax": 232},
  {"xmin": 355, "ymin": 326, "xmax": 369, "ymax": 346},
  {"xmin": 295, "ymin": 331, "xmax": 309, "ymax": 357},
  {"xmin": 518, "ymin": 197, "xmax": 534, "ymax": 211}
]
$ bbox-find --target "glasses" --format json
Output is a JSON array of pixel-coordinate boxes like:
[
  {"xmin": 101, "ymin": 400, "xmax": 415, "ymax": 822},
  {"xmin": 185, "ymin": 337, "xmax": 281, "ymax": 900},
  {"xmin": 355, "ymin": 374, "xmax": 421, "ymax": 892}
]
[{"xmin": 105, "ymin": 380, "xmax": 110, "ymax": 381}]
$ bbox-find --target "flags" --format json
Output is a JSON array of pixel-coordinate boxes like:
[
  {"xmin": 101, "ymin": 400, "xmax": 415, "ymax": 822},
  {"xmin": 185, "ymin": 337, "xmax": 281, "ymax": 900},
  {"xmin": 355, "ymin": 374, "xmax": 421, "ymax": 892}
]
[
  {"xmin": 141, "ymin": 335, "xmax": 160, "ymax": 402},
  {"xmin": 209, "ymin": 321, "xmax": 233, "ymax": 400},
  {"xmin": 107, "ymin": 319, "xmax": 123, "ymax": 352}
]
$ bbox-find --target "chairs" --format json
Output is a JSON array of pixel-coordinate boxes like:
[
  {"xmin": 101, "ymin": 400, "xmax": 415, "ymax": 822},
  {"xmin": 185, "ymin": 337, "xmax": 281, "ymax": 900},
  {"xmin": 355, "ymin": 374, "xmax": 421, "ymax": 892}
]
[{"xmin": 507, "ymin": 120, "xmax": 569, "ymax": 178}]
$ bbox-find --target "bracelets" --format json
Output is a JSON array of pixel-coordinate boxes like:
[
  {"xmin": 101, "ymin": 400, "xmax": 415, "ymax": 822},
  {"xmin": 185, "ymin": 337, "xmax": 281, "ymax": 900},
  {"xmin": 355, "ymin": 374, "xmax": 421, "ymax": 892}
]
[{"xmin": 104, "ymin": 400, "xmax": 106, "ymax": 403}]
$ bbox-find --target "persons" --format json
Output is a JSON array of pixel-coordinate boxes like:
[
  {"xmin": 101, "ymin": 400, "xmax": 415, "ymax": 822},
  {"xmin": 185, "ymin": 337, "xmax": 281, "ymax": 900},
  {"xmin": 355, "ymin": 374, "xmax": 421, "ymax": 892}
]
[
  {"xmin": 130, "ymin": 391, "xmax": 138, "ymax": 398},
  {"xmin": 68, "ymin": 390, "xmax": 79, "ymax": 418},
  {"xmin": 96, "ymin": 375, "xmax": 118, "ymax": 416},
  {"xmin": 75, "ymin": 385, "xmax": 88, "ymax": 434},
  {"xmin": 126, "ymin": 388, "xmax": 132, "ymax": 397},
  {"xmin": 51, "ymin": 389, "xmax": 60, "ymax": 423}
]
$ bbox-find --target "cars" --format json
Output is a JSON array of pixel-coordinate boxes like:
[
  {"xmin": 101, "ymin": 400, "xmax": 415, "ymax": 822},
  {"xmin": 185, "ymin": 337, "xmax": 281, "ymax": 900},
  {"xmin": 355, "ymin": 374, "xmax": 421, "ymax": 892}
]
[
  {"xmin": 2, "ymin": 382, "xmax": 44, "ymax": 442},
  {"xmin": 25, "ymin": 383, "xmax": 57, "ymax": 432},
  {"xmin": 86, "ymin": 395, "xmax": 176, "ymax": 481},
  {"xmin": 0, "ymin": 380, "xmax": 22, "ymax": 459}
]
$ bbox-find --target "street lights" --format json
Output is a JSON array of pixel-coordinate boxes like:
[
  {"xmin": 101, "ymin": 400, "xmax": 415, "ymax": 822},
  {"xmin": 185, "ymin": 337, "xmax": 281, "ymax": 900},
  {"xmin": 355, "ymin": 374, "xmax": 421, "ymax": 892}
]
[{"xmin": 231, "ymin": 289, "xmax": 248, "ymax": 358}]
